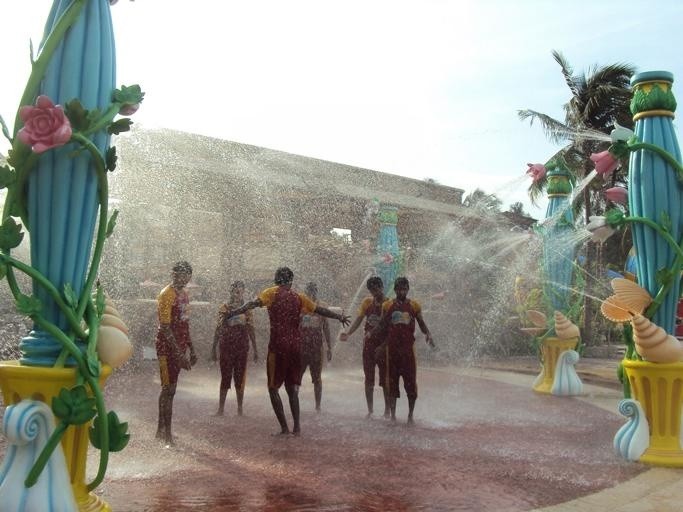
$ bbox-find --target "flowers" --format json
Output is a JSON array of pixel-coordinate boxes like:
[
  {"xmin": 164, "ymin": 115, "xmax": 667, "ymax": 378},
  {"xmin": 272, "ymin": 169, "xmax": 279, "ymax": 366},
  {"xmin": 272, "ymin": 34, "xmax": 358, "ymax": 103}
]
[
  {"xmin": 586, "ymin": 139, "xmax": 683, "ymax": 361},
  {"xmin": 0, "ymin": 4, "xmax": 148, "ymax": 492},
  {"xmin": 524, "ymin": 161, "xmax": 586, "ymax": 324}
]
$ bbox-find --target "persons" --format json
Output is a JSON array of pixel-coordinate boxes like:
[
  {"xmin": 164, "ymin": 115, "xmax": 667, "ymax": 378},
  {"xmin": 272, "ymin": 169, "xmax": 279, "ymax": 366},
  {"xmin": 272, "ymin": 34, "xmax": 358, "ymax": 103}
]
[
  {"xmin": 339, "ymin": 277, "xmax": 391, "ymax": 418},
  {"xmin": 154, "ymin": 260, "xmax": 197, "ymax": 446},
  {"xmin": 218, "ymin": 266, "xmax": 351, "ymax": 436},
  {"xmin": 364, "ymin": 277, "xmax": 435, "ymax": 425},
  {"xmin": 210, "ymin": 280, "xmax": 259, "ymax": 418},
  {"xmin": 294, "ymin": 281, "xmax": 332, "ymax": 410}
]
